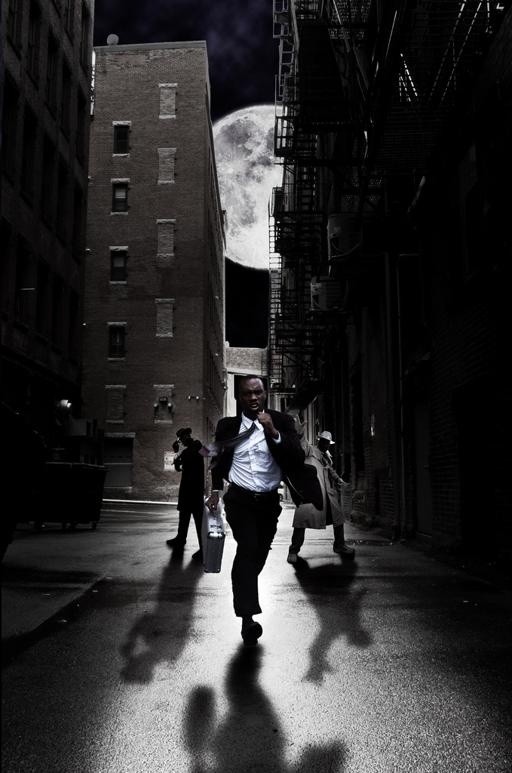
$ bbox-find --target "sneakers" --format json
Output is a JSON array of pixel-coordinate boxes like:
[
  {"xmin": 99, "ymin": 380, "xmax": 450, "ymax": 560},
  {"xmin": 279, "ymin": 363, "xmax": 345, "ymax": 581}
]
[
  {"xmin": 287, "ymin": 553, "xmax": 297, "ymax": 563},
  {"xmin": 333, "ymin": 544, "xmax": 355, "ymax": 555}
]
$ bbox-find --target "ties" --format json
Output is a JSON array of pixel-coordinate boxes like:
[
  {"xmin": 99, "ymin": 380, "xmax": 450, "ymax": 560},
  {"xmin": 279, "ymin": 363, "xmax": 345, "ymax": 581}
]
[{"xmin": 198, "ymin": 422, "xmax": 256, "ymax": 456}]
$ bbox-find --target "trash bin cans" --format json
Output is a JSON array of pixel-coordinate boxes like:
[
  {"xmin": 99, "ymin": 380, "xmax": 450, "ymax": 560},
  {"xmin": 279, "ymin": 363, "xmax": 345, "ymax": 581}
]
[{"xmin": 32, "ymin": 462, "xmax": 112, "ymax": 530}]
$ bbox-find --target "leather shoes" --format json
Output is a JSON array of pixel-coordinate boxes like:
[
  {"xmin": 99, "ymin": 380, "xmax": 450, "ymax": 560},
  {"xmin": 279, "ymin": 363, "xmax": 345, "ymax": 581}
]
[
  {"xmin": 241, "ymin": 620, "xmax": 262, "ymax": 644},
  {"xmin": 192, "ymin": 549, "xmax": 202, "ymax": 560},
  {"xmin": 167, "ymin": 538, "xmax": 185, "ymax": 545}
]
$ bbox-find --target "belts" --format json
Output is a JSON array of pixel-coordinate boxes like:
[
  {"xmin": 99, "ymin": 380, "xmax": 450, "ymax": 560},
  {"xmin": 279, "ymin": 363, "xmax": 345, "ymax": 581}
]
[{"xmin": 251, "ymin": 492, "xmax": 266, "ymax": 499}]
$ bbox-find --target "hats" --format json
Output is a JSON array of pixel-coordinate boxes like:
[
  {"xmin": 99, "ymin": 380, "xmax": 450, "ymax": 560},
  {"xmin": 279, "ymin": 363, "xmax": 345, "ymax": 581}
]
[
  {"xmin": 317, "ymin": 430, "xmax": 335, "ymax": 444},
  {"xmin": 176, "ymin": 428, "xmax": 192, "ymax": 436}
]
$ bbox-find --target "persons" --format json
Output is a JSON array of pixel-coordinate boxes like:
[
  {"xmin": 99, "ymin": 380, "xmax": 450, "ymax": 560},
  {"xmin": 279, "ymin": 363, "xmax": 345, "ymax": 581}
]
[
  {"xmin": 206, "ymin": 456, "xmax": 224, "ymax": 513},
  {"xmin": 288, "ymin": 431, "xmax": 354, "ymax": 563},
  {"xmin": 167, "ymin": 428, "xmax": 204, "ymax": 560},
  {"xmin": 205, "ymin": 375, "xmax": 306, "ymax": 645}
]
